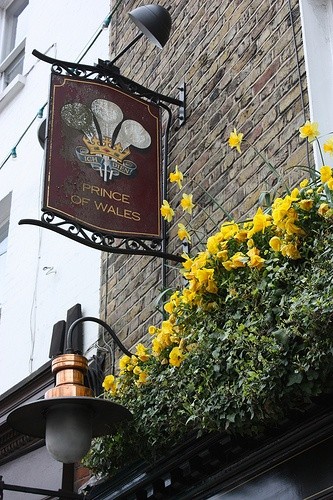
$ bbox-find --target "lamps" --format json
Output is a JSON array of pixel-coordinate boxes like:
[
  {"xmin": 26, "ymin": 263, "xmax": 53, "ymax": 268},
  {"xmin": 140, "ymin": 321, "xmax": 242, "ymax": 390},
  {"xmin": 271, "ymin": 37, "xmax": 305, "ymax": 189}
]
[
  {"xmin": 4, "ymin": 317, "xmax": 139, "ymax": 463},
  {"xmin": 108, "ymin": 5, "xmax": 172, "ymax": 65}
]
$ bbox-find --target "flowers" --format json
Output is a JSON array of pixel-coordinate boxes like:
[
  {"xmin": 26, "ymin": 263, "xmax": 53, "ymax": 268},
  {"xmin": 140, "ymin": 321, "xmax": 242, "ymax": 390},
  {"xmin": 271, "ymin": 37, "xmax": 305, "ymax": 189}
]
[{"xmin": 83, "ymin": 120, "xmax": 333, "ymax": 473}]
